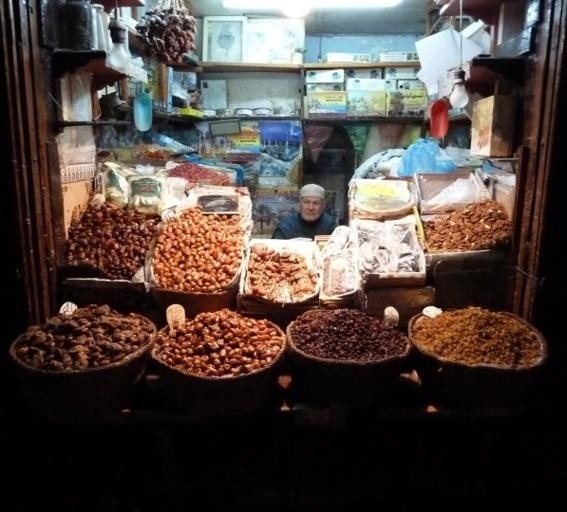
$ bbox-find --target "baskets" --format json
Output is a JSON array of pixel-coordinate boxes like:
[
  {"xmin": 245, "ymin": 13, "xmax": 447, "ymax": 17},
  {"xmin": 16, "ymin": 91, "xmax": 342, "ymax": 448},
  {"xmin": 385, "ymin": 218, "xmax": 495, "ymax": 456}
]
[
  {"xmin": 145, "ymin": 197, "xmax": 251, "ymax": 324},
  {"xmin": 351, "ymin": 174, "xmax": 417, "ymax": 220},
  {"xmin": 59, "ymin": 275, "xmax": 152, "ymax": 313},
  {"xmin": 6, "ymin": 307, "xmax": 159, "ymax": 414},
  {"xmin": 345, "ymin": 215, "xmax": 427, "ymax": 289},
  {"xmin": 236, "ymin": 235, "xmax": 321, "ymax": 317},
  {"xmin": 318, "ymin": 292, "xmax": 363, "ymax": 309},
  {"xmin": 405, "ymin": 302, "xmax": 549, "ymax": 409},
  {"xmin": 153, "ymin": 309, "xmax": 288, "ymax": 415},
  {"xmin": 286, "ymin": 307, "xmax": 414, "ymax": 402}
]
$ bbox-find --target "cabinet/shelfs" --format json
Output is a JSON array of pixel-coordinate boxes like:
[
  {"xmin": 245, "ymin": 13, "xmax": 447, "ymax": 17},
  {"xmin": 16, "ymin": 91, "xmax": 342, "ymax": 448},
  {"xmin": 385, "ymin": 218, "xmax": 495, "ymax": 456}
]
[{"xmin": 37, "ymin": 0, "xmax": 567, "ymax": 125}]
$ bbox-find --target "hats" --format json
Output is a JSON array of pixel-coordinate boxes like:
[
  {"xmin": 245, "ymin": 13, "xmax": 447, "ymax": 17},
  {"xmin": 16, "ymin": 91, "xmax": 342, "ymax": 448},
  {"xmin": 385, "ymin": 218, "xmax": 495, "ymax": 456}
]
[{"xmin": 299, "ymin": 183, "xmax": 326, "ymax": 199}]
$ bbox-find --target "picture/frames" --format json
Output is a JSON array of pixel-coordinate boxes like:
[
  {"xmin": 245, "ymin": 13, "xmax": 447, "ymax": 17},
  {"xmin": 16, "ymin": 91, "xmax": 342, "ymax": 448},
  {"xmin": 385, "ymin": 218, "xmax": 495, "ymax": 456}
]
[{"xmin": 202, "ymin": 16, "xmax": 244, "ymax": 62}]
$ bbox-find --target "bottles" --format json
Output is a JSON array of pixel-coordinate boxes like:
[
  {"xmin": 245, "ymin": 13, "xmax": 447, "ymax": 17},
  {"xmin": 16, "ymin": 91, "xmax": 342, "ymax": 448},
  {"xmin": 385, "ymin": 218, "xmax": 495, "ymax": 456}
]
[{"xmin": 88, "ymin": 4, "xmax": 112, "ymax": 51}]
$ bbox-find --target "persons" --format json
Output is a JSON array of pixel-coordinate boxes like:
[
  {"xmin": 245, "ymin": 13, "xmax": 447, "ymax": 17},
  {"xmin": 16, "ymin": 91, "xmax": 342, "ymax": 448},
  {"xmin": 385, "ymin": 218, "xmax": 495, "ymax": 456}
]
[{"xmin": 271, "ymin": 183, "xmax": 339, "ymax": 239}]
